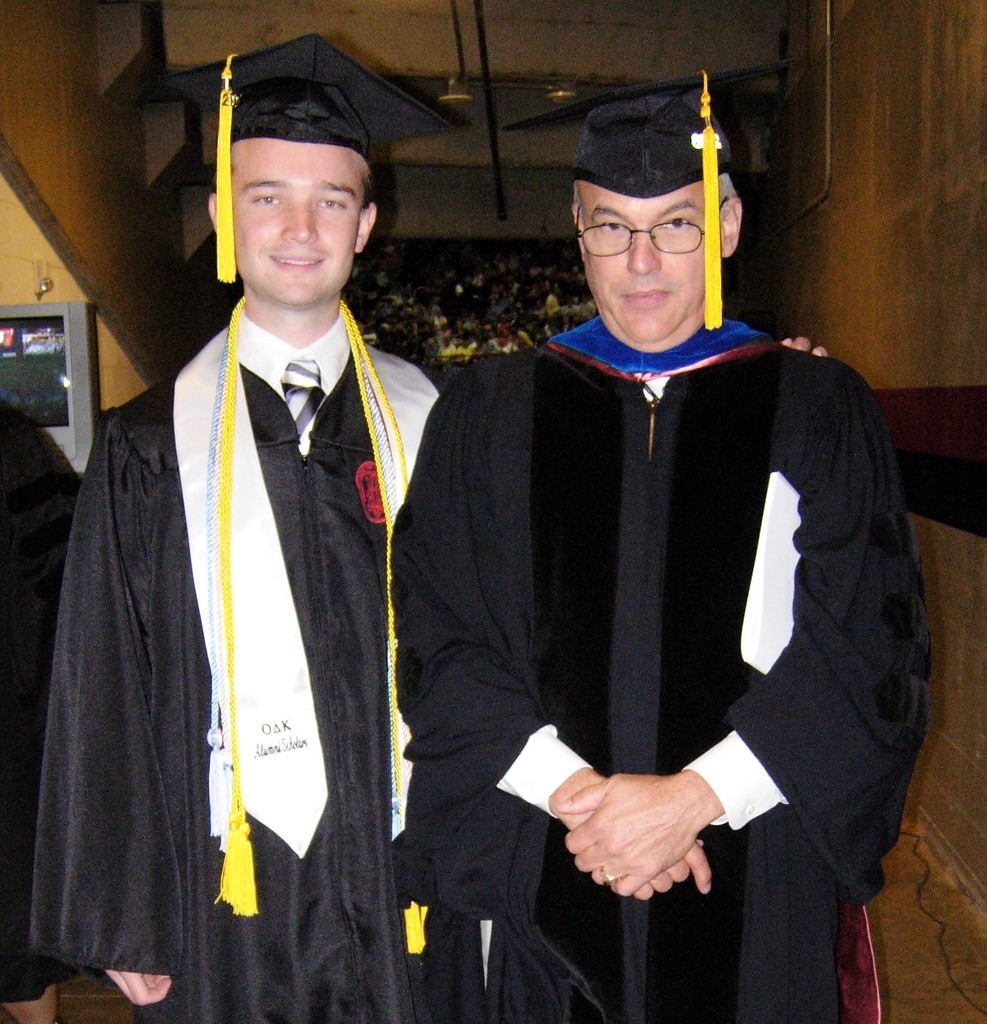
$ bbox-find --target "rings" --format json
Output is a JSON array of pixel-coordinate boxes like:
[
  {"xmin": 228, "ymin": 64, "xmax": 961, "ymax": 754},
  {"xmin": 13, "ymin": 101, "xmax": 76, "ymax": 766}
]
[{"xmin": 599, "ymin": 866, "xmax": 616, "ymax": 887}]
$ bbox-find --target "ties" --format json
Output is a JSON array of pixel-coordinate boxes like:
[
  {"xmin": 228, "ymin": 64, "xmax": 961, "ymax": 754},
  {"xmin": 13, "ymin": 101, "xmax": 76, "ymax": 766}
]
[{"xmin": 282, "ymin": 354, "xmax": 327, "ymax": 457}]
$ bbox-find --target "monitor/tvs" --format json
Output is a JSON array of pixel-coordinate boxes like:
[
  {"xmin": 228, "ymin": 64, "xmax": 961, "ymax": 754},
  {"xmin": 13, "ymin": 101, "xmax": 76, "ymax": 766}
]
[{"xmin": 0, "ymin": 302, "xmax": 101, "ymax": 480}]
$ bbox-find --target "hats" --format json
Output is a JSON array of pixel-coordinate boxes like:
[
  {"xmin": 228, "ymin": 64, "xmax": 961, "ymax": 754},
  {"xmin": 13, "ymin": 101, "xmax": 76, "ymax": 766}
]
[
  {"xmin": 159, "ymin": 32, "xmax": 455, "ymax": 286},
  {"xmin": 503, "ymin": 62, "xmax": 777, "ymax": 332}
]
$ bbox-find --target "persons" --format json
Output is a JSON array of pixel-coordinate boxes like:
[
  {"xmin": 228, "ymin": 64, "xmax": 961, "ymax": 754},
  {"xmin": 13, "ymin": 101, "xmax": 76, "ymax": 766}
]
[
  {"xmin": 352, "ymin": 235, "xmax": 597, "ymax": 364},
  {"xmin": 25, "ymin": 30, "xmax": 833, "ymax": 1023},
  {"xmin": 387, "ymin": 56, "xmax": 929, "ymax": 1024}
]
